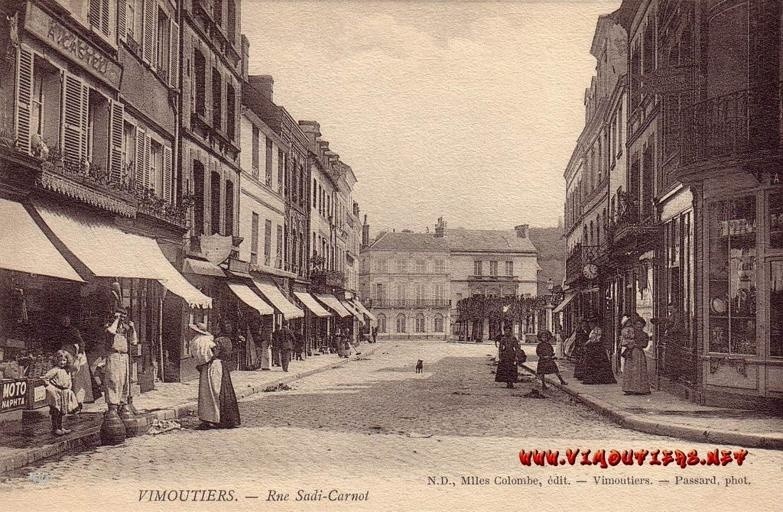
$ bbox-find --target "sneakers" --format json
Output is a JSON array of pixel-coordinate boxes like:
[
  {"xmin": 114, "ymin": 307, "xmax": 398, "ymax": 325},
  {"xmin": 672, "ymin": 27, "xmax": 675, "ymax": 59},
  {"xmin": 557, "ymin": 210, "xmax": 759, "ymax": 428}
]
[{"xmin": 55, "ymin": 428, "xmax": 70, "ymax": 435}]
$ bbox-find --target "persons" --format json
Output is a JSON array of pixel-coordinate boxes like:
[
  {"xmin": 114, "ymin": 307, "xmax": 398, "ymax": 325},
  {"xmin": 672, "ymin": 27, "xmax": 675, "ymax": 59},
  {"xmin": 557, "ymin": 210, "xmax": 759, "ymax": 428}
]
[
  {"xmin": 101, "ymin": 305, "xmax": 139, "ymax": 416},
  {"xmin": 582, "ymin": 316, "xmax": 617, "ymax": 384},
  {"xmin": 274, "ymin": 318, "xmax": 295, "ymax": 373},
  {"xmin": 617, "ymin": 314, "xmax": 634, "ymax": 358},
  {"xmin": 259, "ymin": 323, "xmax": 274, "ymax": 373},
  {"xmin": 337, "ymin": 319, "xmax": 349, "ymax": 360},
  {"xmin": 212, "ymin": 318, "xmax": 243, "ymax": 428},
  {"xmin": 534, "ymin": 330, "xmax": 568, "ymax": 390},
  {"xmin": 619, "ymin": 319, "xmax": 654, "ymax": 395},
  {"xmin": 493, "ymin": 324, "xmax": 525, "ymax": 388},
  {"xmin": 662, "ymin": 304, "xmax": 688, "ymax": 379},
  {"xmin": 50, "ymin": 310, "xmax": 95, "ymax": 405},
  {"xmin": 574, "ymin": 317, "xmax": 589, "ymax": 380},
  {"xmin": 39, "ymin": 348, "xmax": 80, "ymax": 436},
  {"xmin": 370, "ymin": 325, "xmax": 377, "ymax": 344},
  {"xmin": 189, "ymin": 319, "xmax": 221, "ymax": 428}
]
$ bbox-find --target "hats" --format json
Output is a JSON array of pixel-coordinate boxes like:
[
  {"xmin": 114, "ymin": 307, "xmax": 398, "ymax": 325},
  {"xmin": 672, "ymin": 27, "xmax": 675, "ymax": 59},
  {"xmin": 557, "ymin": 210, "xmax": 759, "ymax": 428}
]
[
  {"xmin": 536, "ymin": 330, "xmax": 551, "ymax": 342},
  {"xmin": 188, "ymin": 322, "xmax": 210, "ymax": 336}
]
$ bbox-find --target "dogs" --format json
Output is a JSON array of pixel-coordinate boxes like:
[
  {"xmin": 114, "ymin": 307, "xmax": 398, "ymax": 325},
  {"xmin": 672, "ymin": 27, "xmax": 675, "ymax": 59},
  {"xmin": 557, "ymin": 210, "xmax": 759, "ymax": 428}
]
[{"xmin": 415, "ymin": 359, "xmax": 424, "ymax": 374}]
